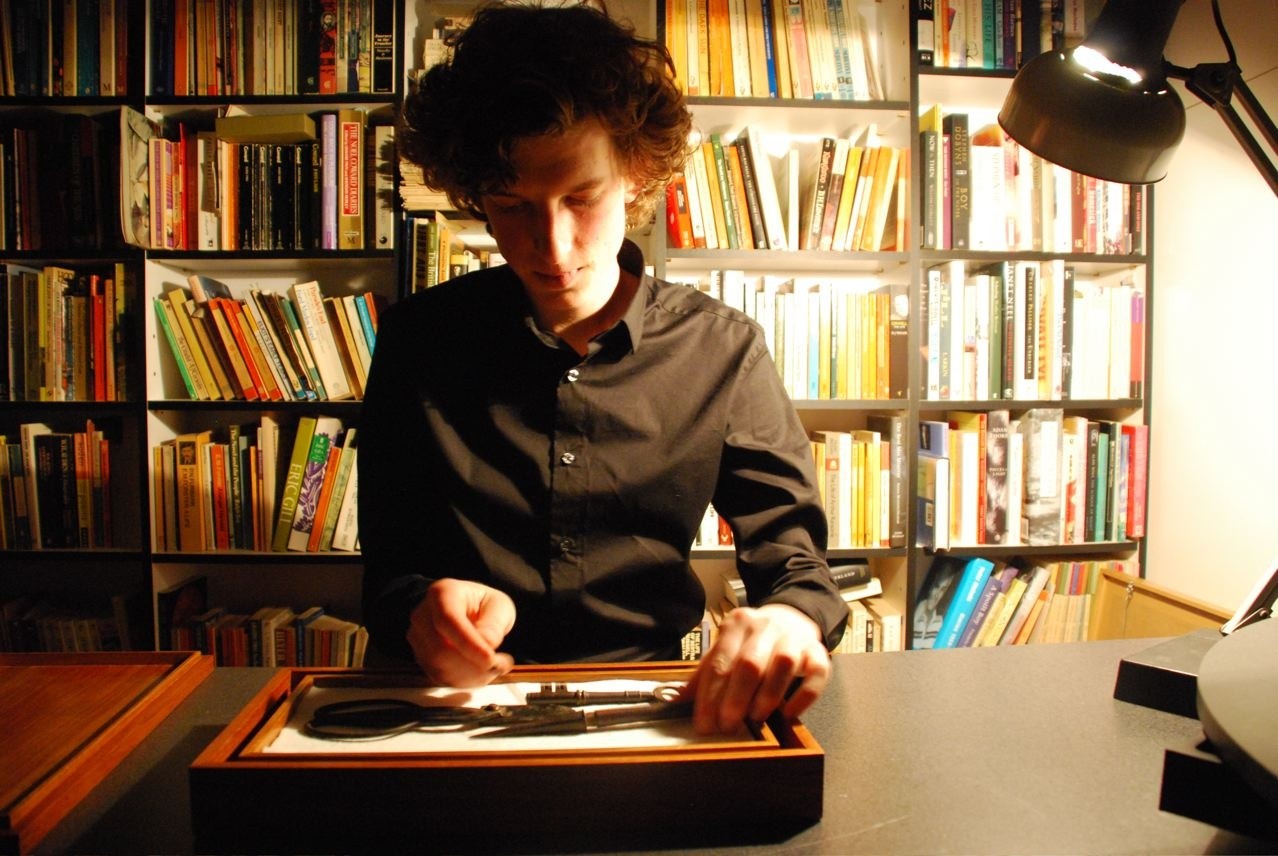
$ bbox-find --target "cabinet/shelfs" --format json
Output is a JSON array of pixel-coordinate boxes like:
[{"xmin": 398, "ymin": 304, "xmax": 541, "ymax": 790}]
[{"xmin": 0, "ymin": 0, "xmax": 1150, "ymax": 672}]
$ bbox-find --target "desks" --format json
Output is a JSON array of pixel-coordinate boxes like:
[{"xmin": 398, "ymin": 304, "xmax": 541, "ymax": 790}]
[{"xmin": 0, "ymin": 637, "xmax": 1278, "ymax": 856}]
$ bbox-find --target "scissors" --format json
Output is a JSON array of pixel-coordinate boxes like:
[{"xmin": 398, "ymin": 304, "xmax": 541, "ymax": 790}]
[{"xmin": 304, "ymin": 697, "xmax": 579, "ymax": 745}]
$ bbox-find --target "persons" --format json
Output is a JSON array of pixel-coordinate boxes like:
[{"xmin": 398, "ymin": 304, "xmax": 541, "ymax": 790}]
[{"xmin": 356, "ymin": 5, "xmax": 850, "ymax": 733}]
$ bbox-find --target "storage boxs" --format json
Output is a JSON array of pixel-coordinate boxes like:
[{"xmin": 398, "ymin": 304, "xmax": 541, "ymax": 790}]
[
  {"xmin": 216, "ymin": 114, "xmax": 317, "ymax": 141},
  {"xmin": 183, "ymin": 664, "xmax": 822, "ymax": 830}
]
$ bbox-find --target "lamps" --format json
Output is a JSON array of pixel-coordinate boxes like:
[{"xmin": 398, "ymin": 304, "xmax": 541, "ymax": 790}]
[{"xmin": 994, "ymin": 0, "xmax": 1278, "ymax": 718}]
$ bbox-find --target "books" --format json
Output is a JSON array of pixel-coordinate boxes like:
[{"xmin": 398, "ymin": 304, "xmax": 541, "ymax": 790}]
[{"xmin": 0, "ymin": 0, "xmax": 1149, "ymax": 669}]
